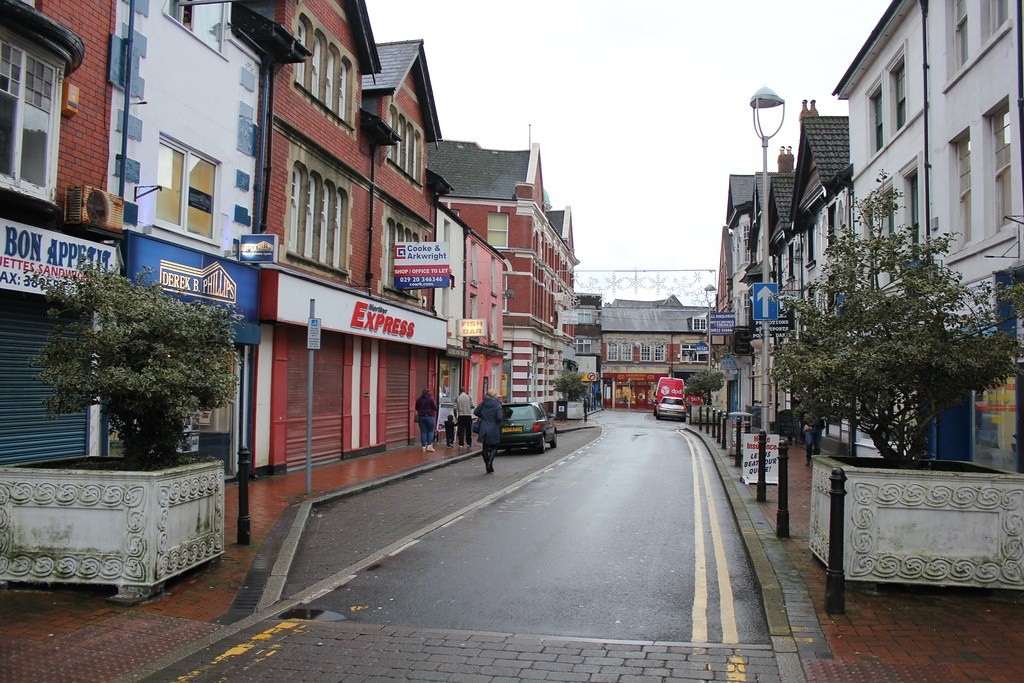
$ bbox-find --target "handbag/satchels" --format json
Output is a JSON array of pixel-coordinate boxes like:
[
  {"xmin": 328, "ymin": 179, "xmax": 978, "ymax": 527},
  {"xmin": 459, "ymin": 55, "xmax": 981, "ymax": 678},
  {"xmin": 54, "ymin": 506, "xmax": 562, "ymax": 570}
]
[
  {"xmin": 414, "ymin": 415, "xmax": 420, "ymax": 422},
  {"xmin": 473, "ymin": 417, "xmax": 480, "ymax": 434}
]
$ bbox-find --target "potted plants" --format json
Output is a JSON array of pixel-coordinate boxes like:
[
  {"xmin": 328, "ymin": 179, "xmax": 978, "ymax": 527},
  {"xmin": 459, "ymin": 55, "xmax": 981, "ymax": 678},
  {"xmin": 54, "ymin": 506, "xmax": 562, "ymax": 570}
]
[
  {"xmin": 764, "ymin": 170, "xmax": 1024, "ymax": 596},
  {"xmin": 553, "ymin": 371, "xmax": 593, "ymax": 420},
  {"xmin": 0, "ymin": 257, "xmax": 243, "ymax": 603}
]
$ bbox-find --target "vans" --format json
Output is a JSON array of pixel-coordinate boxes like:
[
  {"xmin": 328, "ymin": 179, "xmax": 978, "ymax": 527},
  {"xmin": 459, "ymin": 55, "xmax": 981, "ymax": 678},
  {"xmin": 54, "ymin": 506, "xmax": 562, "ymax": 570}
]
[{"xmin": 653, "ymin": 376, "xmax": 684, "ymax": 416}]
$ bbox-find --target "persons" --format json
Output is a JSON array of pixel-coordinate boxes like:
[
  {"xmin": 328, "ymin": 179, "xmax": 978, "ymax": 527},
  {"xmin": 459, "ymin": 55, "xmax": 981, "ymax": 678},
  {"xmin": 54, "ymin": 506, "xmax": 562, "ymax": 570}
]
[
  {"xmin": 415, "ymin": 388, "xmax": 438, "ymax": 452},
  {"xmin": 799, "ymin": 412, "xmax": 825, "ymax": 465},
  {"xmin": 453, "ymin": 387, "xmax": 476, "ymax": 448},
  {"xmin": 596, "ymin": 388, "xmax": 601, "ymax": 406},
  {"xmin": 444, "ymin": 415, "xmax": 458, "ymax": 449},
  {"xmin": 473, "ymin": 389, "xmax": 504, "ymax": 472}
]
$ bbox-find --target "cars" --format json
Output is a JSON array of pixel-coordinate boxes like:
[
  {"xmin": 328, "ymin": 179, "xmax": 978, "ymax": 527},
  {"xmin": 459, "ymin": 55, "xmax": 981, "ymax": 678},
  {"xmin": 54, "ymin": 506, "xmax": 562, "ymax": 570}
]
[
  {"xmin": 655, "ymin": 396, "xmax": 687, "ymax": 422},
  {"xmin": 498, "ymin": 401, "xmax": 557, "ymax": 454}
]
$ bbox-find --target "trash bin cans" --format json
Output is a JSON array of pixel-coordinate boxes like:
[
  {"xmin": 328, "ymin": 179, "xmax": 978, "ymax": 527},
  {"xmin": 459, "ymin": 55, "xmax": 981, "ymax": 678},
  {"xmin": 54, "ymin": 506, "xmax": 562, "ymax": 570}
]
[
  {"xmin": 728, "ymin": 411, "xmax": 753, "ymax": 457},
  {"xmin": 556, "ymin": 397, "xmax": 568, "ymax": 421}
]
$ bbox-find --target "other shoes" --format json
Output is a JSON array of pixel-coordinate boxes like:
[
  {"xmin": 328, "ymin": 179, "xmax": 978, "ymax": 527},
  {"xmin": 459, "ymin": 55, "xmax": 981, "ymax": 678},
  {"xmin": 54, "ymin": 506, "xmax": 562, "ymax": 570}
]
[
  {"xmin": 460, "ymin": 445, "xmax": 463, "ymax": 448},
  {"xmin": 449, "ymin": 443, "xmax": 453, "ymax": 447},
  {"xmin": 467, "ymin": 443, "xmax": 473, "ymax": 447},
  {"xmin": 805, "ymin": 459, "xmax": 810, "ymax": 466},
  {"xmin": 447, "ymin": 445, "xmax": 451, "ymax": 448},
  {"xmin": 427, "ymin": 443, "xmax": 435, "ymax": 452},
  {"xmin": 422, "ymin": 446, "xmax": 426, "ymax": 452},
  {"xmin": 486, "ymin": 466, "xmax": 494, "ymax": 473}
]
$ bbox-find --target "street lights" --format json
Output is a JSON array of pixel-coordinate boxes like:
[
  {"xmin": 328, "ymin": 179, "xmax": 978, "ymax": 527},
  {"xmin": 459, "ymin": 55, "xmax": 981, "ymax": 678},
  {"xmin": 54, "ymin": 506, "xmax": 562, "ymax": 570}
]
[
  {"xmin": 704, "ymin": 285, "xmax": 714, "ymax": 404},
  {"xmin": 748, "ymin": 87, "xmax": 786, "ymax": 503}
]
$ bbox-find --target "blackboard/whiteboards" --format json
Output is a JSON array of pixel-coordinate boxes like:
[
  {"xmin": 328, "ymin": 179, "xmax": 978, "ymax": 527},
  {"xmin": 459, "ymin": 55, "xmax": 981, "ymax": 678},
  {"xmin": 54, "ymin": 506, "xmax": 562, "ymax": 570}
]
[
  {"xmin": 436, "ymin": 403, "xmax": 459, "ymax": 432},
  {"xmin": 777, "ymin": 409, "xmax": 797, "ymax": 437},
  {"xmin": 743, "ymin": 433, "xmax": 780, "ymax": 484}
]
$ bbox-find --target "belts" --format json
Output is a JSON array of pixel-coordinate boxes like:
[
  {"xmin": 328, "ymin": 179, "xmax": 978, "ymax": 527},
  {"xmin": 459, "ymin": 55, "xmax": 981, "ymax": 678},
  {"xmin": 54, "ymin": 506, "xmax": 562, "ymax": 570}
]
[{"xmin": 419, "ymin": 413, "xmax": 432, "ymax": 416}]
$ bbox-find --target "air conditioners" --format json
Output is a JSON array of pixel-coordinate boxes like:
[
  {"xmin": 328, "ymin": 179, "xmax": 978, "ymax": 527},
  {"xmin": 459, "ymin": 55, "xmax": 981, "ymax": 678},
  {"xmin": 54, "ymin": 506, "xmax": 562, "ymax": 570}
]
[{"xmin": 63, "ymin": 185, "xmax": 125, "ymax": 233}]
